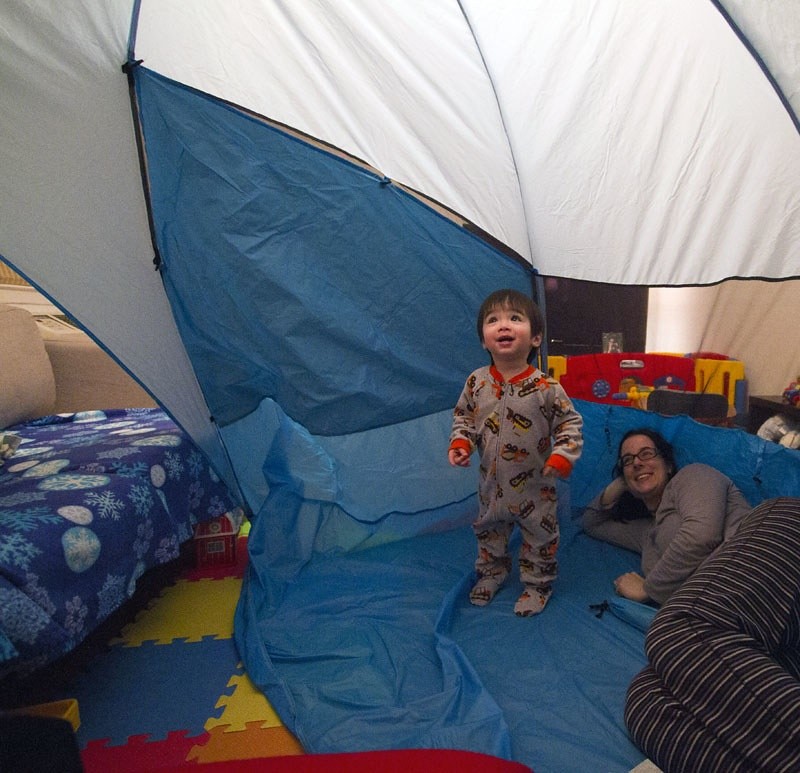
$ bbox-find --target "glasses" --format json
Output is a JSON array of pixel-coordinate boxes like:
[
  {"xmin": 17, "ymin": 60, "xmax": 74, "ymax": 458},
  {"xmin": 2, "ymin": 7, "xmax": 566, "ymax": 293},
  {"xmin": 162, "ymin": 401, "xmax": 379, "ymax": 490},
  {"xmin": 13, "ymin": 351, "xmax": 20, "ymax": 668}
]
[{"xmin": 621, "ymin": 447, "xmax": 662, "ymax": 466}]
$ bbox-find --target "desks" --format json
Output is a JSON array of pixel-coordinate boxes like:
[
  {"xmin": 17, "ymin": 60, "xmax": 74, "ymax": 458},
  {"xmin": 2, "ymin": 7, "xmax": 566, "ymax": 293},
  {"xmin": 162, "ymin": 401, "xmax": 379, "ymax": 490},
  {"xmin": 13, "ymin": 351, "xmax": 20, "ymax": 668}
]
[{"xmin": 748, "ymin": 394, "xmax": 800, "ymax": 445}]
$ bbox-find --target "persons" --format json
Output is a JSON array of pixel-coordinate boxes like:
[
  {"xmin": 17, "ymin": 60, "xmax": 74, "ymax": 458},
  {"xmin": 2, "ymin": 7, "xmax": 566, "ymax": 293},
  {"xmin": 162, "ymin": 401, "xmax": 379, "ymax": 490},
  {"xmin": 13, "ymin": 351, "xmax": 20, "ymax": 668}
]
[
  {"xmin": 447, "ymin": 289, "xmax": 584, "ymax": 617},
  {"xmin": 582, "ymin": 428, "xmax": 800, "ymax": 773}
]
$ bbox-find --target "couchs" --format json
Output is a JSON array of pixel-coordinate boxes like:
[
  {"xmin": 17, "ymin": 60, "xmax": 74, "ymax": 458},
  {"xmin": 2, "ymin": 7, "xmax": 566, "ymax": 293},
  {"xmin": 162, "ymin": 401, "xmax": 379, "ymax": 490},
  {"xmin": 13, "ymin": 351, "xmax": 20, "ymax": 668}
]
[{"xmin": 0, "ymin": 303, "xmax": 234, "ymax": 678}]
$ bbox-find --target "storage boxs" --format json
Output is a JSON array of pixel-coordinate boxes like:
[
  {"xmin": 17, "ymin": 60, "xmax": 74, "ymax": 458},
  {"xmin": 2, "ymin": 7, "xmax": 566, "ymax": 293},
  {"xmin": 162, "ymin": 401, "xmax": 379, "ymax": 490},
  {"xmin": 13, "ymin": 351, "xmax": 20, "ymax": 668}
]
[{"xmin": 182, "ymin": 515, "xmax": 238, "ymax": 568}]
